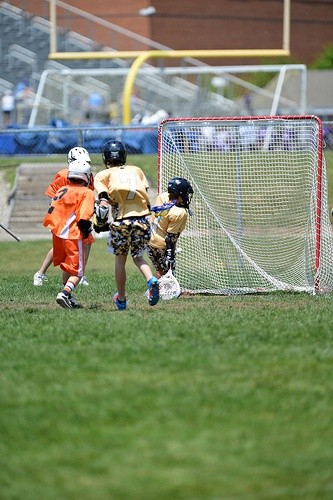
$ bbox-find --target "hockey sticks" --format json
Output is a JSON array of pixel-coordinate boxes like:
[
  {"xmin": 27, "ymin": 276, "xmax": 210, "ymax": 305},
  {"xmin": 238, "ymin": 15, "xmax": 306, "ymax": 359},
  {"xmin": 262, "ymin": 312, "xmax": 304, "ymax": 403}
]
[
  {"xmin": 145, "ymin": 262, "xmax": 182, "ymax": 300},
  {"xmin": 0, "ymin": 223, "xmax": 21, "ymax": 243}
]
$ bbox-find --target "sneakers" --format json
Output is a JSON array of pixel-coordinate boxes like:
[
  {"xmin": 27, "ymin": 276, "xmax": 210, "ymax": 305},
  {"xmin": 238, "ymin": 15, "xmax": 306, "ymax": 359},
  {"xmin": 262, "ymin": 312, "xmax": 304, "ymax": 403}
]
[
  {"xmin": 33, "ymin": 272, "xmax": 48, "ymax": 287},
  {"xmin": 78, "ymin": 276, "xmax": 89, "ymax": 286},
  {"xmin": 147, "ymin": 276, "xmax": 160, "ymax": 306},
  {"xmin": 113, "ymin": 292, "xmax": 127, "ymax": 310},
  {"xmin": 55, "ymin": 292, "xmax": 74, "ymax": 310},
  {"xmin": 69, "ymin": 299, "xmax": 79, "ymax": 309}
]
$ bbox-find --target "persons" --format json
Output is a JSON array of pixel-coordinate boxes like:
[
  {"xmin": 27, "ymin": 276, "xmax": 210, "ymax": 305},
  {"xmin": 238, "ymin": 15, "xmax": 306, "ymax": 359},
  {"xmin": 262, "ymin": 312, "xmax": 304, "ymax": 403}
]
[
  {"xmin": 92, "ymin": 140, "xmax": 160, "ymax": 311},
  {"xmin": 33, "ymin": 146, "xmax": 95, "ymax": 288},
  {"xmin": 2, "ymin": 71, "xmax": 333, "ymax": 156},
  {"xmin": 146, "ymin": 178, "xmax": 194, "ymax": 299},
  {"xmin": 44, "ymin": 161, "xmax": 96, "ymax": 312}
]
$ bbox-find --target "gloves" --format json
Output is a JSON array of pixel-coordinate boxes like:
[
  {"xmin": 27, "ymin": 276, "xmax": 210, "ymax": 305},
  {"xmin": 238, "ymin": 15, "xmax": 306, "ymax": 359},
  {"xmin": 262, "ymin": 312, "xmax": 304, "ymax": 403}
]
[{"xmin": 166, "ymin": 248, "xmax": 175, "ymax": 269}]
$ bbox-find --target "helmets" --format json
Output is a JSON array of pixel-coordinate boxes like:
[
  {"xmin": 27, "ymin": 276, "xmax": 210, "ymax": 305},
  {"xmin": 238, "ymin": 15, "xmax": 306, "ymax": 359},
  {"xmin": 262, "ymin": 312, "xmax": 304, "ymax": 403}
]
[
  {"xmin": 67, "ymin": 146, "xmax": 92, "ymax": 163},
  {"xmin": 167, "ymin": 177, "xmax": 194, "ymax": 206},
  {"xmin": 102, "ymin": 140, "xmax": 126, "ymax": 166},
  {"xmin": 66, "ymin": 159, "xmax": 91, "ymax": 184}
]
